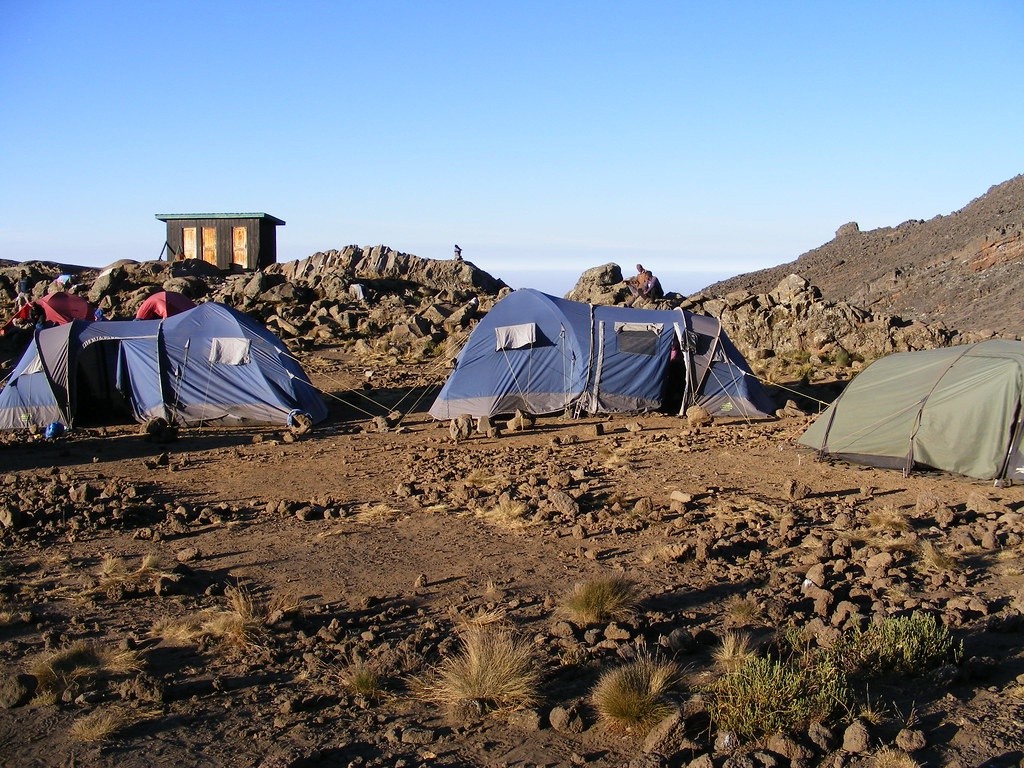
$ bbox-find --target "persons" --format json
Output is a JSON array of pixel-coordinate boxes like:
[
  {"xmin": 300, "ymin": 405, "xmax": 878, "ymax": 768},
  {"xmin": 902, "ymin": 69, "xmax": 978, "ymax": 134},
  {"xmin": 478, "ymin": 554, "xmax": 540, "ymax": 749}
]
[
  {"xmin": 642, "ymin": 271, "xmax": 664, "ymax": 303},
  {"xmin": 12, "ymin": 317, "xmax": 36, "ymax": 330},
  {"xmin": 17, "ymin": 269, "xmax": 36, "ymax": 312},
  {"xmin": 27, "ymin": 301, "xmax": 60, "ymax": 330},
  {"xmin": 94, "ymin": 308, "xmax": 110, "ymax": 322},
  {"xmin": 634, "ymin": 264, "xmax": 650, "ymax": 292}
]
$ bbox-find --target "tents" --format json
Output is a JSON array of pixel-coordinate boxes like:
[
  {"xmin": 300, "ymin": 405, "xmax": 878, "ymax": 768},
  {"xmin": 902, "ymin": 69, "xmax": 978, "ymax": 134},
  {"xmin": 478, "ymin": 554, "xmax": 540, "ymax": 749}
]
[
  {"xmin": 429, "ymin": 287, "xmax": 774, "ymax": 422},
  {"xmin": 796, "ymin": 337, "xmax": 1023, "ymax": 485},
  {"xmin": 0, "ymin": 291, "xmax": 110, "ymax": 335},
  {"xmin": 136, "ymin": 291, "xmax": 196, "ymax": 321},
  {"xmin": 0, "ymin": 300, "xmax": 328, "ymax": 431}
]
